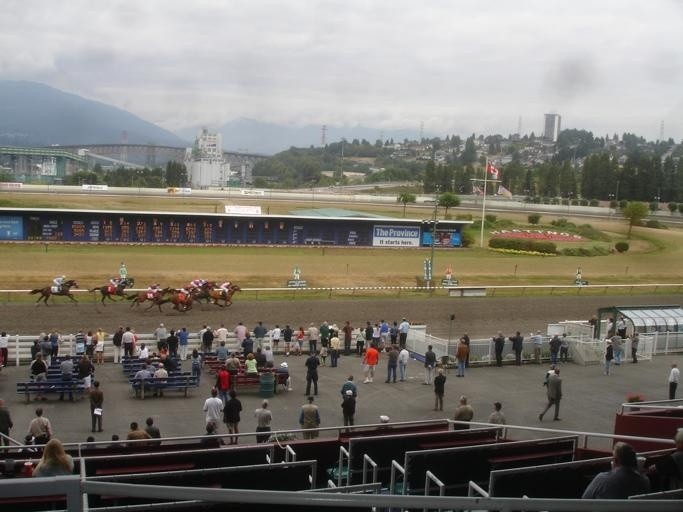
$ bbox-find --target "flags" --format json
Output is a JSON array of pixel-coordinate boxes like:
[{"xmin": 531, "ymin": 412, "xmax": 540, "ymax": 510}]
[{"xmin": 487, "ymin": 157, "xmax": 499, "ymax": 177}]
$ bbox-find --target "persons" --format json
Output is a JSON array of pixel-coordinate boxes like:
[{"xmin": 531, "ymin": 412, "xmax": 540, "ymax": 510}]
[
  {"xmin": 424, "ymin": 333, "xmax": 470, "ymax": 411},
  {"xmin": 655, "ymin": 427, "xmax": 683, "ymax": 490},
  {"xmin": 454, "ymin": 396, "xmax": 473, "ymax": 430},
  {"xmin": 604, "ymin": 314, "xmax": 639, "ymax": 376},
  {"xmin": 487, "ymin": 401, "xmax": 506, "ymax": 436},
  {"xmin": 376, "ymin": 416, "xmax": 392, "ymax": 430},
  {"xmin": 113, "ymin": 317, "xmax": 410, "ymax": 396},
  {"xmin": 199, "ymin": 389, "xmax": 241, "ymax": 449},
  {"xmin": 581, "ymin": 441, "xmax": 650, "ymax": 499},
  {"xmin": 530, "ymin": 330, "xmax": 542, "ymax": 364},
  {"xmin": 560, "ymin": 333, "xmax": 569, "ymax": 364},
  {"xmin": 89, "ymin": 381, "xmax": 105, "ymax": 432},
  {"xmin": 341, "ymin": 390, "xmax": 355, "ymax": 432},
  {"xmin": 254, "ymin": 399, "xmax": 272, "ymax": 442},
  {"xmin": 549, "ymin": 335, "xmax": 561, "ymax": 367},
  {"xmin": 299, "ymin": 397, "xmax": 320, "ymax": 439},
  {"xmin": 0, "ymin": 401, "xmax": 74, "ymax": 477},
  {"xmin": 509, "ymin": 331, "xmax": 523, "ymax": 366},
  {"xmin": 0, "ymin": 332, "xmax": 10, "ymax": 367},
  {"xmin": 493, "ymin": 331, "xmax": 506, "ymax": 367},
  {"xmin": 539, "ymin": 364, "xmax": 562, "ymax": 421},
  {"xmin": 51, "ymin": 265, "xmax": 233, "ymax": 302},
  {"xmin": 29, "ymin": 327, "xmax": 109, "ymax": 401},
  {"xmin": 576, "ymin": 264, "xmax": 582, "ymax": 280},
  {"xmin": 87, "ymin": 418, "xmax": 162, "ymax": 449},
  {"xmin": 668, "ymin": 364, "xmax": 681, "ymax": 399}
]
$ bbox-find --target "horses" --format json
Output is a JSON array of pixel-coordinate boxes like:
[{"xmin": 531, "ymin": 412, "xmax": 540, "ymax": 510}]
[
  {"xmin": 27, "ymin": 279, "xmax": 82, "ymax": 307},
  {"xmin": 127, "ymin": 281, "xmax": 242, "ymax": 313},
  {"xmin": 88, "ymin": 277, "xmax": 136, "ymax": 305}
]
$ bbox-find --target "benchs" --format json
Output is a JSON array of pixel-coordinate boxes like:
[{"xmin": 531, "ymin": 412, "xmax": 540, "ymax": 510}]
[
  {"xmin": 13, "ymin": 349, "xmax": 292, "ymax": 407},
  {"xmin": 0, "ymin": 417, "xmax": 683, "ymax": 511}
]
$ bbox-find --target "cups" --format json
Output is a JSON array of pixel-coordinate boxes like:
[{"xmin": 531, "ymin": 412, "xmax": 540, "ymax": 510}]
[{"xmin": 23, "ymin": 462, "xmax": 32, "ymax": 476}]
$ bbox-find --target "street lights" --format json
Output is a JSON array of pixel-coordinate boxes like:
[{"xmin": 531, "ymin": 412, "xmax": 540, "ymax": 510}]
[
  {"xmin": 432, "ymin": 199, "xmax": 440, "ymax": 276},
  {"xmin": 609, "ymin": 193, "xmax": 616, "ymax": 210},
  {"xmin": 566, "ymin": 191, "xmax": 573, "ymax": 213},
  {"xmin": 451, "ymin": 179, "xmax": 456, "ymax": 194},
  {"xmin": 523, "ymin": 189, "xmax": 529, "ymax": 212},
  {"xmin": 616, "ymin": 180, "xmax": 620, "ymax": 207},
  {"xmin": 655, "ymin": 196, "xmax": 661, "ymax": 215},
  {"xmin": 435, "ymin": 184, "xmax": 443, "ymax": 200}
]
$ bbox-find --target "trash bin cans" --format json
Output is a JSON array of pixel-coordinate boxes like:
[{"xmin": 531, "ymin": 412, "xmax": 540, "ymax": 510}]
[{"xmin": 258, "ymin": 372, "xmax": 275, "ymax": 398}]
